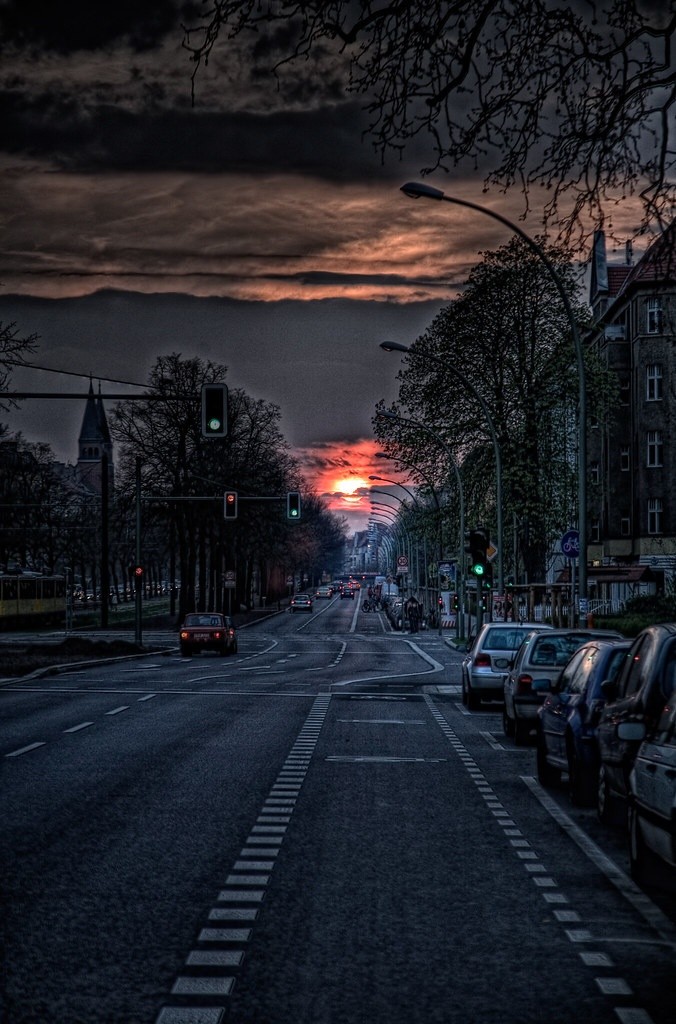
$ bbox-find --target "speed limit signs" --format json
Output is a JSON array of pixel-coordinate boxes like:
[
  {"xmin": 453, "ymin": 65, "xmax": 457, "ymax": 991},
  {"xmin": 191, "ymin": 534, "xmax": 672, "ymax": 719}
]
[
  {"xmin": 398, "ymin": 556, "xmax": 408, "ymax": 567},
  {"xmin": 225, "ymin": 570, "xmax": 234, "ymax": 581}
]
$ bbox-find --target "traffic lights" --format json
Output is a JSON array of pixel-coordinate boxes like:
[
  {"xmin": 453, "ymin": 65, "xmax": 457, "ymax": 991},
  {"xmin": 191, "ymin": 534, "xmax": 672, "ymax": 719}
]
[
  {"xmin": 224, "ymin": 492, "xmax": 238, "ymax": 519},
  {"xmin": 201, "ymin": 384, "xmax": 229, "ymax": 437},
  {"xmin": 134, "ymin": 566, "xmax": 143, "ymax": 590},
  {"xmin": 287, "ymin": 492, "xmax": 301, "ymax": 520},
  {"xmin": 470, "ymin": 529, "xmax": 489, "ymax": 577}
]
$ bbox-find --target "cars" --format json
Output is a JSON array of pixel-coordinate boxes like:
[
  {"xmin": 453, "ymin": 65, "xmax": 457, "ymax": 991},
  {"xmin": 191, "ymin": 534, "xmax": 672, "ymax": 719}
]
[
  {"xmin": 67, "ymin": 582, "xmax": 174, "ymax": 603},
  {"xmin": 290, "ymin": 595, "xmax": 312, "ymax": 613},
  {"xmin": 179, "ymin": 613, "xmax": 237, "ymax": 658},
  {"xmin": 315, "ymin": 579, "xmax": 360, "ymax": 600},
  {"xmin": 462, "ymin": 623, "xmax": 676, "ymax": 882},
  {"xmin": 363, "ymin": 584, "xmax": 426, "ymax": 631}
]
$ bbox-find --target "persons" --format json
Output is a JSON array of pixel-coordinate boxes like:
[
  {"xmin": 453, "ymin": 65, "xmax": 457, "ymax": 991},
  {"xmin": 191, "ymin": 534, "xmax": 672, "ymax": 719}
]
[
  {"xmin": 407, "ymin": 602, "xmax": 419, "ymax": 635},
  {"xmin": 367, "ymin": 586, "xmax": 379, "ymax": 613},
  {"xmin": 109, "ymin": 587, "xmax": 118, "ymax": 612}
]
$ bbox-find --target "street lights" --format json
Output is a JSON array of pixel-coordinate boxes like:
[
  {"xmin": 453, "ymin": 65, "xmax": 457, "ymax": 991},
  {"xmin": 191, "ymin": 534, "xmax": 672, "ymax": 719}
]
[
  {"xmin": 376, "ymin": 409, "xmax": 462, "ymax": 574},
  {"xmin": 370, "ymin": 476, "xmax": 428, "ymax": 586},
  {"xmin": 371, "ymin": 502, "xmax": 419, "ymax": 583},
  {"xmin": 400, "ymin": 182, "xmax": 587, "ymax": 628},
  {"xmin": 374, "ymin": 452, "xmax": 442, "ymax": 559},
  {"xmin": 380, "ymin": 340, "xmax": 504, "ymax": 595}
]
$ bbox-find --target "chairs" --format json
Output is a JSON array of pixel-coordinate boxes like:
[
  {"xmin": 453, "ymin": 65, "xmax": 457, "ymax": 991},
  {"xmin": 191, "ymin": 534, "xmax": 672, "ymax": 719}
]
[
  {"xmin": 538, "ymin": 644, "xmax": 557, "ymax": 666},
  {"xmin": 210, "ymin": 618, "xmax": 219, "ymax": 625},
  {"xmin": 489, "ymin": 635, "xmax": 507, "ymax": 648}
]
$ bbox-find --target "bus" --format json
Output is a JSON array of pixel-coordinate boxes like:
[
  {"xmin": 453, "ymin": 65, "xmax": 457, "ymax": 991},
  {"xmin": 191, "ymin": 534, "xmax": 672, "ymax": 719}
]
[{"xmin": 0, "ymin": 569, "xmax": 67, "ymax": 631}]
